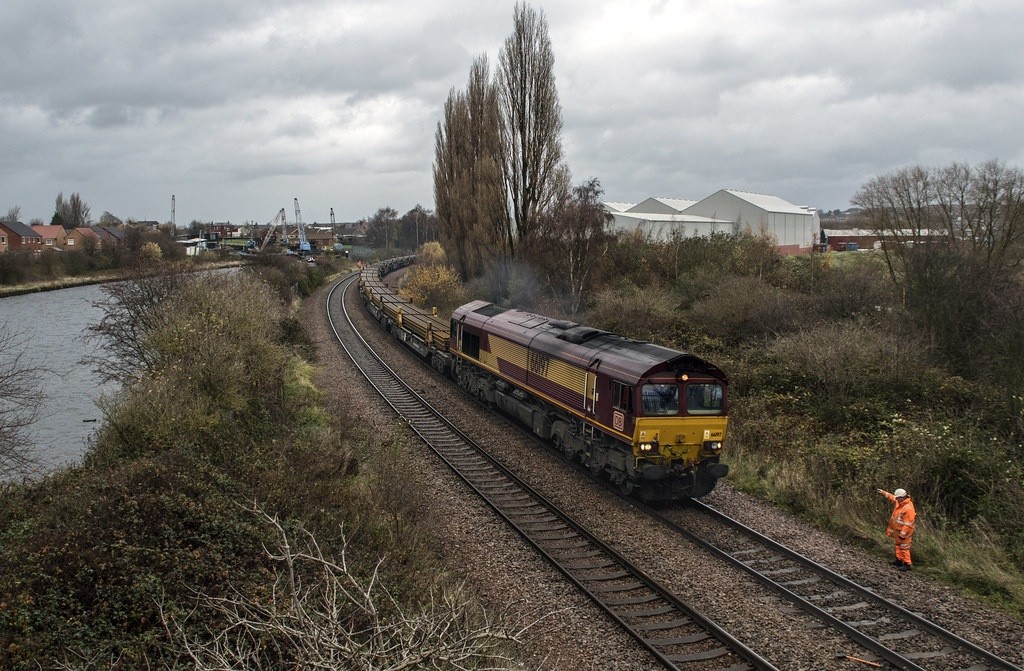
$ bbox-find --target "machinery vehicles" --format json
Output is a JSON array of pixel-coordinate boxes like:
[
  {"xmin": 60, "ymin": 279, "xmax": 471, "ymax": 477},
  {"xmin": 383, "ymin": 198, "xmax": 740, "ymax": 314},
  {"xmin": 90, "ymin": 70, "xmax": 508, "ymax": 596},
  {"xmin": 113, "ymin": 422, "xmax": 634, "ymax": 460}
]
[
  {"xmin": 294, "ymin": 197, "xmax": 311, "ymax": 255},
  {"xmin": 280, "ymin": 208, "xmax": 289, "ymax": 246}
]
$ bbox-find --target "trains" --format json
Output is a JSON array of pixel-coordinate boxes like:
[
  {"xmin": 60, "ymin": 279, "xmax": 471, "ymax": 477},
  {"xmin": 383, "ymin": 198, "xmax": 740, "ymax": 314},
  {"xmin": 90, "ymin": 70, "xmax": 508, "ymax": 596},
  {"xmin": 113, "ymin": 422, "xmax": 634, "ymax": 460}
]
[{"xmin": 356, "ymin": 252, "xmax": 731, "ymax": 508}]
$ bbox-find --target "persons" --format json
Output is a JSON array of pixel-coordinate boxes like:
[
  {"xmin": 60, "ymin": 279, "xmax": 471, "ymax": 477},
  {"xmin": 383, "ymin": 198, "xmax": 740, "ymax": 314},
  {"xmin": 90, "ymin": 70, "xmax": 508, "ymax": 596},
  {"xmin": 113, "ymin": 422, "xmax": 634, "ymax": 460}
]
[{"xmin": 877, "ymin": 488, "xmax": 916, "ymax": 572}]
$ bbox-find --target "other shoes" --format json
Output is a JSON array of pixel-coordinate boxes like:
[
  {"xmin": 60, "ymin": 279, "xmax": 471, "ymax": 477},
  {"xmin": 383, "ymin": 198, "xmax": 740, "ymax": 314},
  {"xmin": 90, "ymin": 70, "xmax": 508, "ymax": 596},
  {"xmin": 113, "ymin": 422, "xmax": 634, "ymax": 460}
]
[
  {"xmin": 890, "ymin": 559, "xmax": 902, "ymax": 566},
  {"xmin": 899, "ymin": 565, "xmax": 913, "ymax": 571}
]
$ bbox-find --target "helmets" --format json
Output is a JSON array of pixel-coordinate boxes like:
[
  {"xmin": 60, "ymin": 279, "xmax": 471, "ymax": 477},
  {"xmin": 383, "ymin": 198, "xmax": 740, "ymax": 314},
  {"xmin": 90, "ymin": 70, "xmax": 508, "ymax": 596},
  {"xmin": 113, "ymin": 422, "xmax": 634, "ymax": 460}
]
[{"xmin": 893, "ymin": 488, "xmax": 907, "ymax": 498}]
